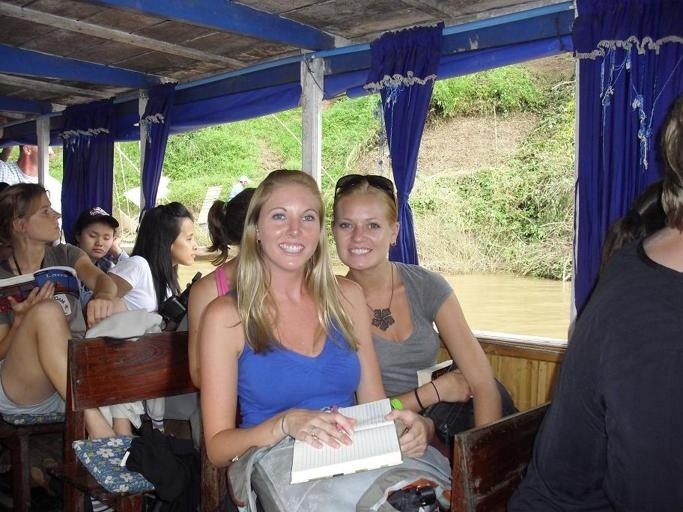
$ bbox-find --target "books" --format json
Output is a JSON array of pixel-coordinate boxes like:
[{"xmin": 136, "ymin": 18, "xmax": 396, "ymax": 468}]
[
  {"xmin": 290, "ymin": 397, "xmax": 403, "ymax": 484},
  {"xmin": 0, "ymin": 265, "xmax": 81, "ymax": 323}
]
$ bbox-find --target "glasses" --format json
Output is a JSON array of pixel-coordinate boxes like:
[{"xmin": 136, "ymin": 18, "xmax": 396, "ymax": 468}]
[{"xmin": 334, "ymin": 173, "xmax": 395, "ymax": 204}]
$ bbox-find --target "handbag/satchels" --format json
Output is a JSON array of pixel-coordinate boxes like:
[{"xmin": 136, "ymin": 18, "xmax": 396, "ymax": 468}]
[
  {"xmin": 421, "ymin": 358, "xmax": 521, "ymax": 449},
  {"xmin": 226, "ymin": 416, "xmax": 454, "ymax": 511}
]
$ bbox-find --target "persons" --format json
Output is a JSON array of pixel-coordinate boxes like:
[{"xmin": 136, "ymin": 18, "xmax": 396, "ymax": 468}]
[
  {"xmin": 103, "ymin": 200, "xmax": 203, "ymax": 455},
  {"xmin": 504, "ymin": 93, "xmax": 683, "ymax": 511},
  {"xmin": 229, "ymin": 174, "xmax": 248, "ymax": 200},
  {"xmin": 0, "ymin": 141, "xmax": 64, "ymax": 246},
  {"xmin": 0, "ymin": 182, "xmax": 143, "ymax": 442},
  {"xmin": 195, "ymin": 167, "xmax": 436, "ymax": 511},
  {"xmin": 329, "ymin": 173, "xmax": 523, "ymax": 454},
  {"xmin": 69, "ymin": 204, "xmax": 129, "ymax": 316},
  {"xmin": 184, "ymin": 187, "xmax": 257, "ymax": 392}
]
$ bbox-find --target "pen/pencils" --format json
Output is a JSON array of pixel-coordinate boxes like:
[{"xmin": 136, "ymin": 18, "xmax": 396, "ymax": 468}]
[{"xmin": 330, "ymin": 404, "xmax": 342, "ymax": 431}]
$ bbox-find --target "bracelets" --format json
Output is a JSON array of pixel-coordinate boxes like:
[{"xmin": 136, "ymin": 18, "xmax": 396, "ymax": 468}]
[
  {"xmin": 280, "ymin": 407, "xmax": 288, "ymax": 435},
  {"xmin": 413, "ymin": 387, "xmax": 425, "ymax": 411},
  {"xmin": 429, "ymin": 380, "xmax": 441, "ymax": 403}
]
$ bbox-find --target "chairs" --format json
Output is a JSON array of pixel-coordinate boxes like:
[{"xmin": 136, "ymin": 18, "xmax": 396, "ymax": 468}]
[{"xmin": 57, "ymin": 329, "xmax": 220, "ymax": 511}]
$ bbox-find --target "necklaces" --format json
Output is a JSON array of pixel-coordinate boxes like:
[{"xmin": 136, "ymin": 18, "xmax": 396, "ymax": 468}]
[{"xmin": 343, "ymin": 263, "xmax": 398, "ymax": 333}]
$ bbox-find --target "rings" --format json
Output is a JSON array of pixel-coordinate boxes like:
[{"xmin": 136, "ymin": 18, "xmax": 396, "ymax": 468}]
[{"xmin": 310, "ymin": 424, "xmax": 319, "ymax": 440}]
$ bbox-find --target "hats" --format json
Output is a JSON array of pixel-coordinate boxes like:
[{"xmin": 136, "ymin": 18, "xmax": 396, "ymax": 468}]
[{"xmin": 77, "ymin": 206, "xmax": 119, "ymax": 234}]
